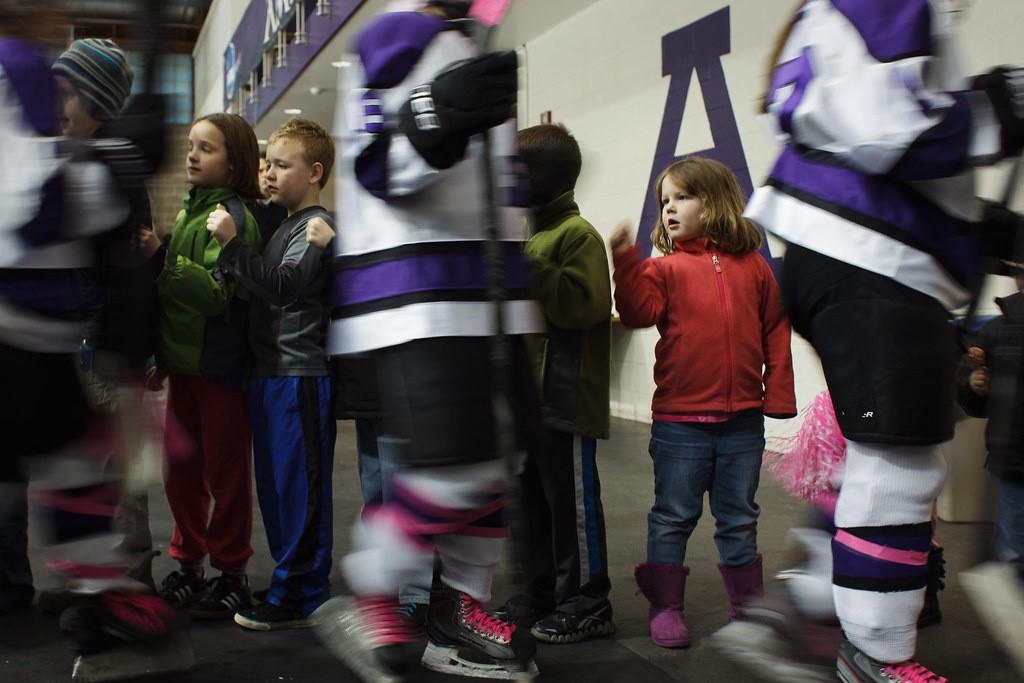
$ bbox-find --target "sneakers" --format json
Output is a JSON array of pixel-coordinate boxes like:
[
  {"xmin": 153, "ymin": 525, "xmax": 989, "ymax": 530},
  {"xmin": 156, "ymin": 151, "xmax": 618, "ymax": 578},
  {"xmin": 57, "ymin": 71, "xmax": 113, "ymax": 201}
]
[
  {"xmin": 423, "ymin": 584, "xmax": 542, "ymax": 680},
  {"xmin": 189, "ymin": 575, "xmax": 252, "ymax": 619},
  {"xmin": 836, "ymin": 628, "xmax": 951, "ymax": 683},
  {"xmin": 314, "ymin": 591, "xmax": 414, "ymax": 682},
  {"xmin": 491, "ymin": 596, "xmax": 548, "ymax": 627},
  {"xmin": 530, "ymin": 599, "xmax": 617, "ymax": 643},
  {"xmin": 160, "ymin": 567, "xmax": 208, "ymax": 620}
]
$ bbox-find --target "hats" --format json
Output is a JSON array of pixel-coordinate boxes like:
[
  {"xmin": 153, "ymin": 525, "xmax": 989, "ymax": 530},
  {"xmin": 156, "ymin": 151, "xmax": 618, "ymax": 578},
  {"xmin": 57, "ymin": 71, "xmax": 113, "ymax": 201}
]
[{"xmin": 51, "ymin": 38, "xmax": 135, "ymax": 122}]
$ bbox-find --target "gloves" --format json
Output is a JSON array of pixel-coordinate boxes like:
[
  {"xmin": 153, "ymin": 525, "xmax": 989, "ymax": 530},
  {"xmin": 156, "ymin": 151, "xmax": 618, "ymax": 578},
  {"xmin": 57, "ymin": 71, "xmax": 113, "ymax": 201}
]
[
  {"xmin": 399, "ymin": 50, "xmax": 518, "ymax": 169},
  {"xmin": 972, "ymin": 67, "xmax": 1024, "ymax": 157}
]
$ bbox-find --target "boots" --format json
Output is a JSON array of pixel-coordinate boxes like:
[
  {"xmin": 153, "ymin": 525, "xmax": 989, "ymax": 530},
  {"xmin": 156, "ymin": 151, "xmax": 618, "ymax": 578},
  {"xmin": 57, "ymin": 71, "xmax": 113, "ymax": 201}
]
[
  {"xmin": 716, "ymin": 553, "xmax": 764, "ymax": 622},
  {"xmin": 635, "ymin": 562, "xmax": 692, "ymax": 647}
]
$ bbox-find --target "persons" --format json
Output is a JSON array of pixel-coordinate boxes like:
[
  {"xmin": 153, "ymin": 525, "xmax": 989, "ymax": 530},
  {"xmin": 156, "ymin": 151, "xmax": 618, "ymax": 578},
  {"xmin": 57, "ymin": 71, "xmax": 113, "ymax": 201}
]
[
  {"xmin": 305, "ymin": 217, "xmax": 426, "ymax": 638},
  {"xmin": 710, "ymin": 0, "xmax": 1024, "ymax": 683},
  {"xmin": 45, "ymin": 38, "xmax": 162, "ymax": 594},
  {"xmin": 245, "ymin": 150, "xmax": 288, "ymax": 243},
  {"xmin": 506, "ymin": 123, "xmax": 616, "ymax": 642},
  {"xmin": 0, "ymin": 36, "xmax": 102, "ymax": 644},
  {"xmin": 310, "ymin": 0, "xmax": 540, "ymax": 683},
  {"xmin": 207, "ymin": 115, "xmax": 336, "ymax": 631},
  {"xmin": 609, "ymin": 156, "xmax": 798, "ymax": 646},
  {"xmin": 954, "ymin": 279, "xmax": 1024, "ymax": 578},
  {"xmin": 131, "ymin": 113, "xmax": 263, "ymax": 619}
]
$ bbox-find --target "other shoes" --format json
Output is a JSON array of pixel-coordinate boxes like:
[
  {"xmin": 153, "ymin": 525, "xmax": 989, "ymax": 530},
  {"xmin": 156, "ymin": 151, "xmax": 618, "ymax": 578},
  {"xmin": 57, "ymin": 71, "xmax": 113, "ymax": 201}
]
[
  {"xmin": 234, "ymin": 579, "xmax": 331, "ymax": 630},
  {"xmin": 58, "ymin": 578, "xmax": 153, "ymax": 639},
  {"xmin": 38, "ymin": 576, "xmax": 84, "ymax": 623}
]
